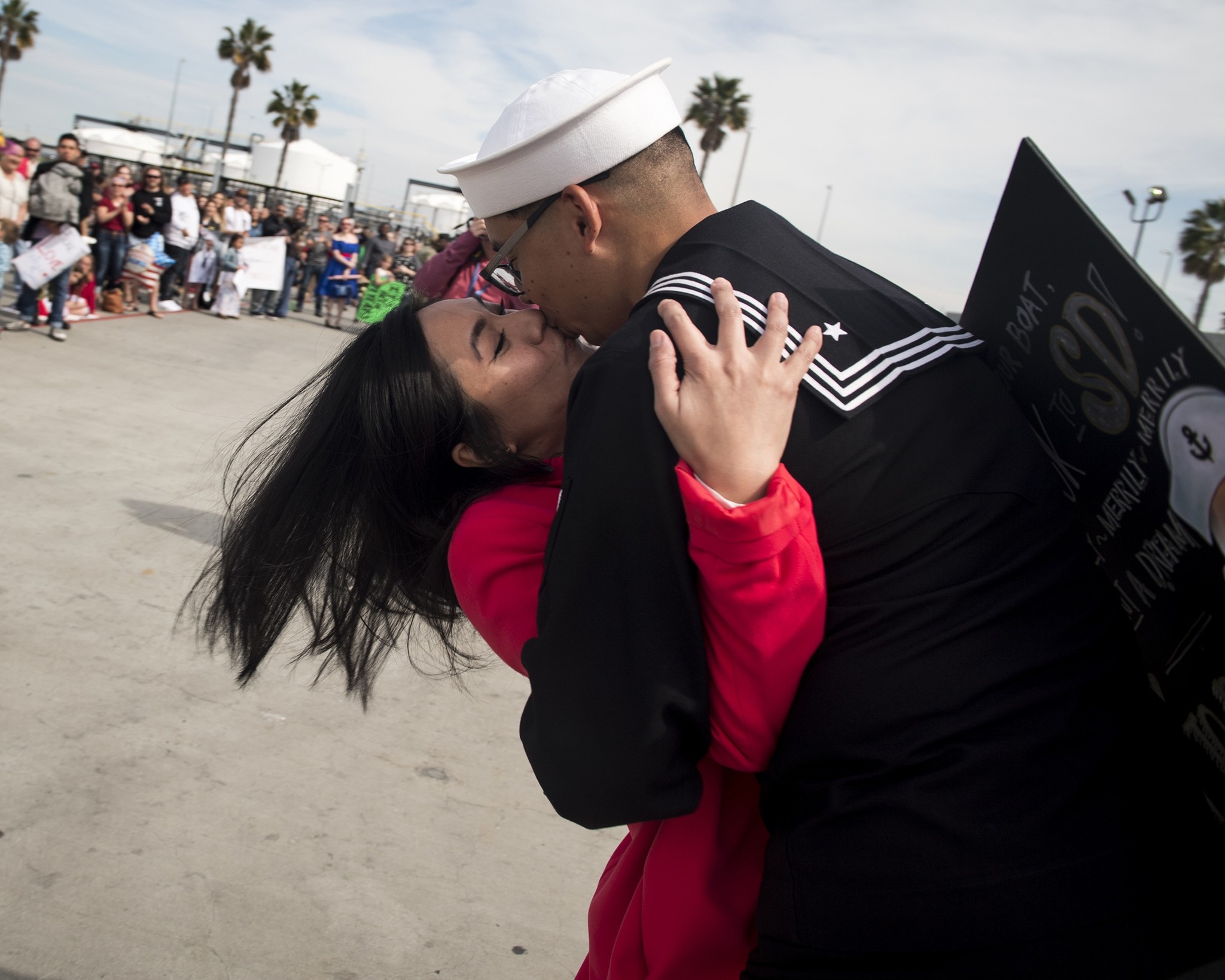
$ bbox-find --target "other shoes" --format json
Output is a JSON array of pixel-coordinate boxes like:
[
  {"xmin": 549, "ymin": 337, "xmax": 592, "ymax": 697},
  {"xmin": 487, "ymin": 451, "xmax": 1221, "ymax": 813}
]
[
  {"xmin": 182, "ymin": 300, "xmax": 287, "ymax": 321},
  {"xmin": 324, "ymin": 319, "xmax": 342, "ymax": 329},
  {"xmin": 52, "ymin": 327, "xmax": 67, "ymax": 341},
  {"xmin": 293, "ymin": 306, "xmax": 302, "ymax": 311},
  {"xmin": 315, "ymin": 309, "xmax": 323, "ymax": 317},
  {"xmin": 4, "ymin": 321, "xmax": 29, "ymax": 329}
]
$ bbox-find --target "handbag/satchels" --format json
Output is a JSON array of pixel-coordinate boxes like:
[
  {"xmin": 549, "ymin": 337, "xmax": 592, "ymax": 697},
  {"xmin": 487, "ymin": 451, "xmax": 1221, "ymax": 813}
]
[
  {"xmin": 334, "ymin": 280, "xmax": 353, "ymax": 296},
  {"xmin": 104, "ymin": 289, "xmax": 125, "ymax": 312}
]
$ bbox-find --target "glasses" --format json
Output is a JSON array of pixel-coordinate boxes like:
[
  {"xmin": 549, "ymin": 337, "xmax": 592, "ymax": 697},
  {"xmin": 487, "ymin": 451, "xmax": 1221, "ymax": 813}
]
[
  {"xmin": 146, "ymin": 174, "xmax": 159, "ymax": 180},
  {"xmin": 236, "ymin": 192, "xmax": 248, "ymax": 199},
  {"xmin": 111, "ymin": 184, "xmax": 126, "ymax": 188},
  {"xmin": 480, "ymin": 171, "xmax": 610, "ymax": 295},
  {"xmin": 23, "ymin": 146, "xmax": 39, "ymax": 153}
]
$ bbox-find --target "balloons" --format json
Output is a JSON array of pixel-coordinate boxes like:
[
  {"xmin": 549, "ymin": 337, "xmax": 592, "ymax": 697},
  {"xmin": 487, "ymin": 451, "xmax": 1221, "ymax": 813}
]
[{"xmin": 116, "ymin": 231, "xmax": 176, "ymax": 293}]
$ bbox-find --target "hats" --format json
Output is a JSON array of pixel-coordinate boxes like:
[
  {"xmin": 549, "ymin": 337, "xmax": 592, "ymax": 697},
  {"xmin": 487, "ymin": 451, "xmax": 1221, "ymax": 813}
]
[
  {"xmin": 175, "ymin": 176, "xmax": 190, "ymax": 185},
  {"xmin": 437, "ymin": 56, "xmax": 681, "ymax": 219}
]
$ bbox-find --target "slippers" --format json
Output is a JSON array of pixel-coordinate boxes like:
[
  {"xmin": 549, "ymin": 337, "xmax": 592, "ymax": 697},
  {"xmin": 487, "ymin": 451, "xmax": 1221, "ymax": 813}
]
[
  {"xmin": 147, "ymin": 308, "xmax": 164, "ymax": 319},
  {"xmin": 126, "ymin": 305, "xmax": 139, "ymax": 312}
]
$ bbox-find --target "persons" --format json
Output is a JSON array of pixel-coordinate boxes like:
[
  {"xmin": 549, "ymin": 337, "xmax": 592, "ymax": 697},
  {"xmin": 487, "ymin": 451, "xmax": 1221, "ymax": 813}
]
[
  {"xmin": 438, "ymin": 58, "xmax": 1225, "ymax": 980},
  {"xmin": 243, "ymin": 204, "xmax": 359, "ymax": 330},
  {"xmin": 0, "ymin": 133, "xmax": 92, "ymax": 343},
  {"xmin": 173, "ymin": 278, "xmax": 825, "ymax": 980},
  {"xmin": 412, "ymin": 219, "xmax": 539, "ymax": 311},
  {"xmin": 414, "ymin": 233, "xmax": 453, "ymax": 263},
  {"xmin": 78, "ymin": 149, "xmax": 252, "ymax": 318},
  {"xmin": 353, "ymin": 223, "xmax": 423, "ymax": 322}
]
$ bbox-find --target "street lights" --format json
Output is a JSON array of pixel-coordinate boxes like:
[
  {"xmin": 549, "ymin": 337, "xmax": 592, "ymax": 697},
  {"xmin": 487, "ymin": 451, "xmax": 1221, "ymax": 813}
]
[{"xmin": 1121, "ymin": 185, "xmax": 1170, "ymax": 259}]
